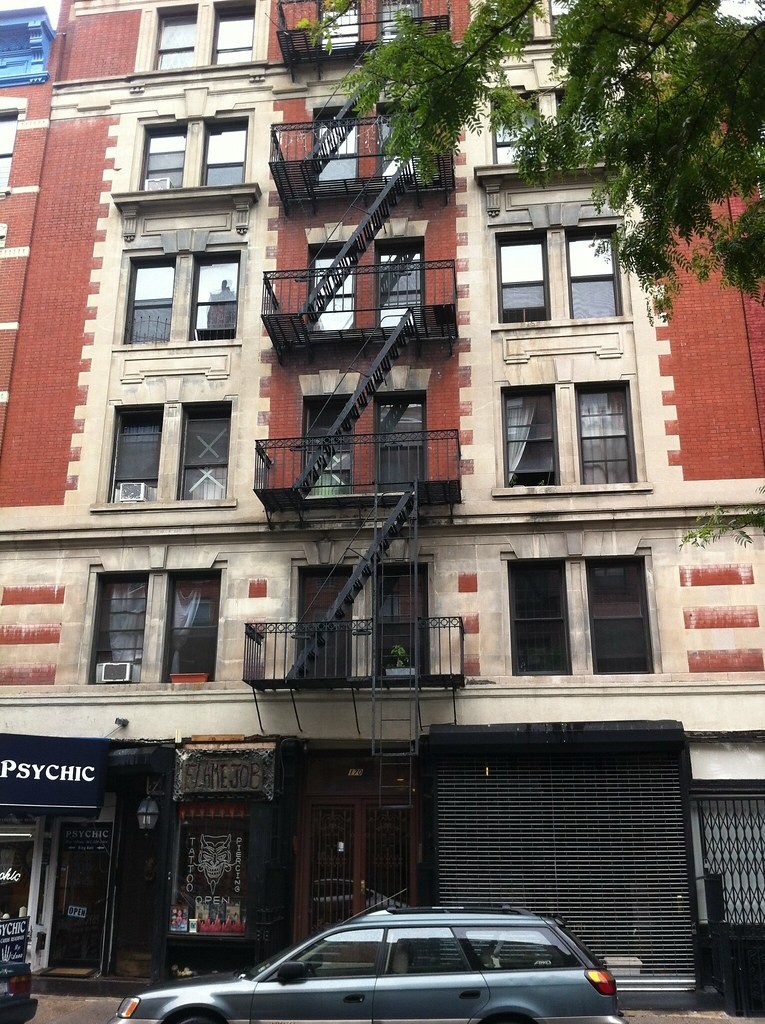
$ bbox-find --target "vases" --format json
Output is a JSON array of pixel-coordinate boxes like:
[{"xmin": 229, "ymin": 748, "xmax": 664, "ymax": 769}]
[{"xmin": 171, "ymin": 673, "xmax": 209, "ymax": 682}]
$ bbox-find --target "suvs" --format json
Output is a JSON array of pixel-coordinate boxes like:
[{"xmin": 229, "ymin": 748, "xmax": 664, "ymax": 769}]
[{"xmin": 105, "ymin": 906, "xmax": 628, "ymax": 1024}]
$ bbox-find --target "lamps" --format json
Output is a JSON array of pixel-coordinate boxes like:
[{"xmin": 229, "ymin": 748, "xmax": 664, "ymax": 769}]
[{"xmin": 137, "ymin": 797, "xmax": 159, "ymax": 830}]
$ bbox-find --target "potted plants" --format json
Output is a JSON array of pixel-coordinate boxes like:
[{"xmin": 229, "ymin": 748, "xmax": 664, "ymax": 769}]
[{"xmin": 385, "ymin": 644, "xmax": 415, "ymax": 676}]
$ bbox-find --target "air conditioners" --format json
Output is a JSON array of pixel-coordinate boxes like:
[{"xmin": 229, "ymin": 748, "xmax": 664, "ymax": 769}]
[
  {"xmin": 119, "ymin": 482, "xmax": 150, "ymax": 502},
  {"xmin": 145, "ymin": 178, "xmax": 175, "ymax": 190},
  {"xmin": 102, "ymin": 662, "xmax": 132, "ymax": 682}
]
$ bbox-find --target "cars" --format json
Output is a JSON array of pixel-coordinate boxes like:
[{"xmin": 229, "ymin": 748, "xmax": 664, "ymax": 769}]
[
  {"xmin": 0, "ymin": 960, "xmax": 38, "ymax": 1024},
  {"xmin": 313, "ymin": 879, "xmax": 409, "ymax": 918}
]
